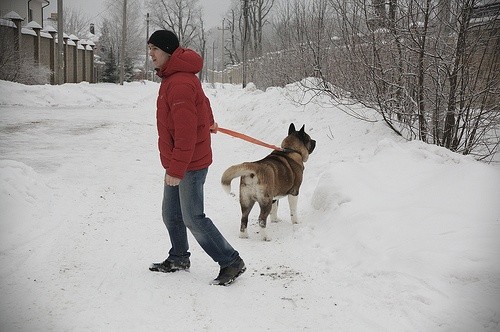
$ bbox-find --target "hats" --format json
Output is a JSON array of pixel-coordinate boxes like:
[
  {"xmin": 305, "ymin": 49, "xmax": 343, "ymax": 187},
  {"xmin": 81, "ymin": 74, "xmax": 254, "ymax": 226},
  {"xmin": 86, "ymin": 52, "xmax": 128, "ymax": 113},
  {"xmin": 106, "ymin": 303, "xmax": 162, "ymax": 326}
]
[{"xmin": 147, "ymin": 30, "xmax": 180, "ymax": 55}]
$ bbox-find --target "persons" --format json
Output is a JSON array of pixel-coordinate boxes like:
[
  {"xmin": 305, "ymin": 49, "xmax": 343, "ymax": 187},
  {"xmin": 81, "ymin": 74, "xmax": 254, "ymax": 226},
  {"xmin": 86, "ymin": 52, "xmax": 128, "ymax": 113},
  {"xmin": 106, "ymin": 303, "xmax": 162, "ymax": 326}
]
[{"xmin": 148, "ymin": 29, "xmax": 247, "ymax": 286}]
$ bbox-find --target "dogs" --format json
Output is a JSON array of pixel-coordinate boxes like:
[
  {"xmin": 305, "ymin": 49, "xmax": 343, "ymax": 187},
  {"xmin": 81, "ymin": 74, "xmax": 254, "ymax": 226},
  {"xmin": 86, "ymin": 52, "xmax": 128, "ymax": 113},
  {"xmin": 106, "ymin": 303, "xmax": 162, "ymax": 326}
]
[{"xmin": 223, "ymin": 123, "xmax": 317, "ymax": 240}]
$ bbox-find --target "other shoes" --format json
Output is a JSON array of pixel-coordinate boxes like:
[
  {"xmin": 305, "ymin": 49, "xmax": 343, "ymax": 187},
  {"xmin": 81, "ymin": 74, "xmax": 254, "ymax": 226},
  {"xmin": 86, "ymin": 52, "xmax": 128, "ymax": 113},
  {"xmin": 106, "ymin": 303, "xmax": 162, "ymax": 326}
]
[{"xmin": 149, "ymin": 254, "xmax": 247, "ymax": 287}]
